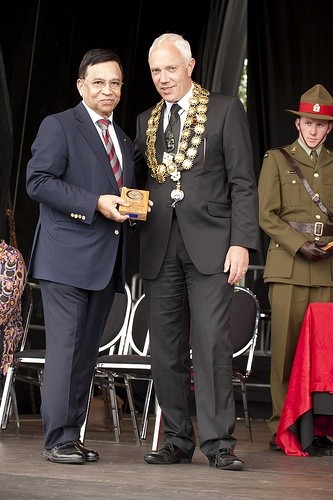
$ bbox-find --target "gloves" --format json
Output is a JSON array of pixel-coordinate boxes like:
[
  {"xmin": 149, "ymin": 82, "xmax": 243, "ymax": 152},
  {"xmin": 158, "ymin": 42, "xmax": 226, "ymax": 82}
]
[{"xmin": 298, "ymin": 241, "xmax": 331, "ymax": 262}]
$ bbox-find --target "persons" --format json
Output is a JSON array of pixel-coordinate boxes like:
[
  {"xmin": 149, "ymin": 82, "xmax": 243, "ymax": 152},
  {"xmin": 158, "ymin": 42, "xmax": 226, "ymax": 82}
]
[
  {"xmin": 24, "ymin": 49, "xmax": 153, "ymax": 464},
  {"xmin": 135, "ymin": 33, "xmax": 261, "ymax": 470},
  {"xmin": 254, "ymin": 85, "xmax": 333, "ymax": 455}
]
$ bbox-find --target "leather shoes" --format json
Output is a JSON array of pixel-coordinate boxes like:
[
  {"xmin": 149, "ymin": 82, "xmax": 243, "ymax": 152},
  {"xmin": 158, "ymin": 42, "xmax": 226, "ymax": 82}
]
[
  {"xmin": 42, "ymin": 441, "xmax": 85, "ymax": 464},
  {"xmin": 269, "ymin": 433, "xmax": 280, "ymax": 449},
  {"xmin": 73, "ymin": 440, "xmax": 99, "ymax": 461},
  {"xmin": 313, "ymin": 435, "xmax": 333, "ymax": 455},
  {"xmin": 208, "ymin": 447, "xmax": 244, "ymax": 470},
  {"xmin": 144, "ymin": 443, "xmax": 192, "ymax": 465}
]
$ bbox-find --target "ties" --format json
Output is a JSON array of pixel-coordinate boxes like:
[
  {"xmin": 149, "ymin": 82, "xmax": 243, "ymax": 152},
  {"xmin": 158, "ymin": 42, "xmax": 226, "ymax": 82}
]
[
  {"xmin": 95, "ymin": 119, "xmax": 124, "ymax": 197},
  {"xmin": 164, "ymin": 104, "xmax": 182, "ymax": 161}
]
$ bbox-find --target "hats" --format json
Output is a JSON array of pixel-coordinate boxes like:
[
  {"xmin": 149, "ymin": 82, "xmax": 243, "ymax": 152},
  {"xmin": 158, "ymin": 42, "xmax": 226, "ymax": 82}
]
[{"xmin": 288, "ymin": 84, "xmax": 333, "ymax": 120}]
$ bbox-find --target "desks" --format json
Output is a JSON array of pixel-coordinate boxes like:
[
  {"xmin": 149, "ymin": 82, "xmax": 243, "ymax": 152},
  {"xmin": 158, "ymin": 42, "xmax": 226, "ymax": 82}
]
[{"xmin": 275, "ymin": 303, "xmax": 333, "ymax": 456}]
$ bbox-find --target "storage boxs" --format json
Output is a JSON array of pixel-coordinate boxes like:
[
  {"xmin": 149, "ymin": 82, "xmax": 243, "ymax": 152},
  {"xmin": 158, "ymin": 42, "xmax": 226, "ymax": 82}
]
[{"xmin": 119, "ymin": 188, "xmax": 150, "ymax": 220}]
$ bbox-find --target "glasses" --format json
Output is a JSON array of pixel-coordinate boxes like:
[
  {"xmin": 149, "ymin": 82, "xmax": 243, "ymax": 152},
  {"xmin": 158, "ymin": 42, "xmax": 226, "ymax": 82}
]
[{"xmin": 83, "ymin": 80, "xmax": 124, "ymax": 89}]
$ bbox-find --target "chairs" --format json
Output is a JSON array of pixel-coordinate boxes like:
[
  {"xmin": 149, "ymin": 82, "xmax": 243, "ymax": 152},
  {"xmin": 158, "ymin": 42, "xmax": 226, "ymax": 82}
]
[{"xmin": 0, "ymin": 284, "xmax": 260, "ymax": 452}]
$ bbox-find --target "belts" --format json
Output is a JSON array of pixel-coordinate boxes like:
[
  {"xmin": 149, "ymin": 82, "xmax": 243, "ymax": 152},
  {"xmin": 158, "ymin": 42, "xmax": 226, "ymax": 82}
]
[{"xmin": 287, "ymin": 221, "xmax": 333, "ymax": 237}]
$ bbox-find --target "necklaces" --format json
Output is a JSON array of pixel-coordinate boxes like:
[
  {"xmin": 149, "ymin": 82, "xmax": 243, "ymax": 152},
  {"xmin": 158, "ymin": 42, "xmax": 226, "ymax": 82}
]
[{"xmin": 145, "ymin": 78, "xmax": 210, "ymax": 206}]
